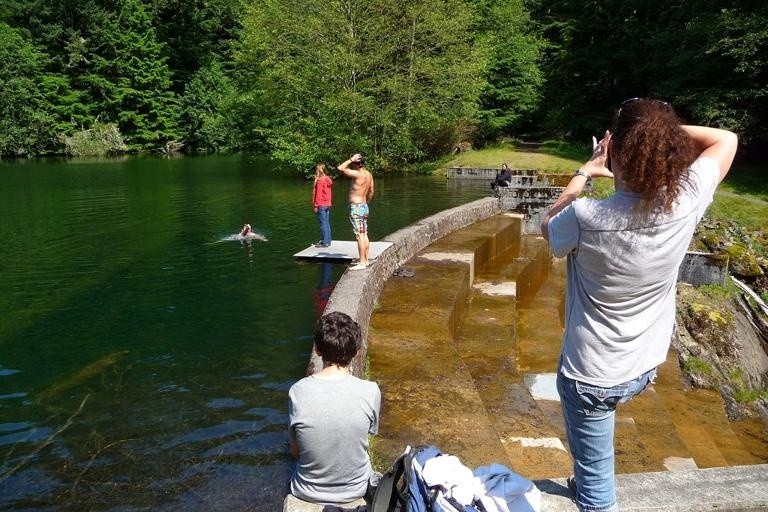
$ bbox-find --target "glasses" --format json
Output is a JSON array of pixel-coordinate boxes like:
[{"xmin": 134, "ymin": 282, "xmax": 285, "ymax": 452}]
[{"xmin": 618, "ymin": 97, "xmax": 668, "ymax": 115}]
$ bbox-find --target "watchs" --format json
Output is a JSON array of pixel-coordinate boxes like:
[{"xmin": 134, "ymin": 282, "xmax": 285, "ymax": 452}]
[{"xmin": 574, "ymin": 169, "xmax": 592, "ymax": 181}]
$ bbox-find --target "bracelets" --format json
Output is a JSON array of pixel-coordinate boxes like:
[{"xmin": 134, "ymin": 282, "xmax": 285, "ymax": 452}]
[{"xmin": 349, "ymin": 158, "xmax": 353, "ymax": 162}]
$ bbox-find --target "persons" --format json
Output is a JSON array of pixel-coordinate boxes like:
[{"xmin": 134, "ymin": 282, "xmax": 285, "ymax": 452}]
[
  {"xmin": 311, "ymin": 164, "xmax": 333, "ymax": 247},
  {"xmin": 336, "ymin": 153, "xmax": 374, "ymax": 270},
  {"xmin": 491, "ymin": 163, "xmax": 511, "ymax": 190},
  {"xmin": 287, "ymin": 311, "xmax": 381, "ymax": 504},
  {"xmin": 314, "ymin": 262, "xmax": 336, "ymax": 312},
  {"xmin": 541, "ymin": 99, "xmax": 742, "ymax": 512}
]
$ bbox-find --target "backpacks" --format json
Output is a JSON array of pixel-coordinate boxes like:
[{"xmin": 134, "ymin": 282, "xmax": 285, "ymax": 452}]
[{"xmin": 371, "ymin": 443, "xmax": 540, "ymax": 512}]
